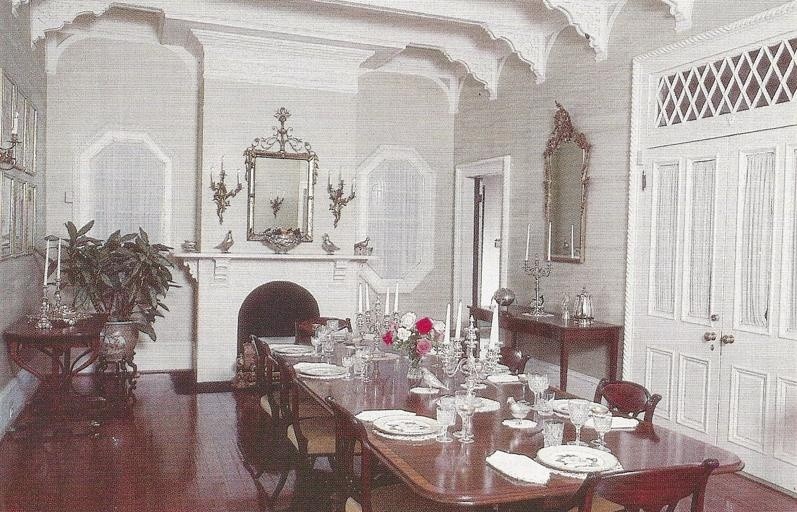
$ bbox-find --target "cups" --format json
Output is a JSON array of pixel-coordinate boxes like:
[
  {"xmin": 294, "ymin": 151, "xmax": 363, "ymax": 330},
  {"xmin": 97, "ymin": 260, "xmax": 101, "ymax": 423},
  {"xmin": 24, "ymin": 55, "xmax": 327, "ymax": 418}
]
[{"xmin": 541, "ymin": 418, "xmax": 565, "ymax": 446}]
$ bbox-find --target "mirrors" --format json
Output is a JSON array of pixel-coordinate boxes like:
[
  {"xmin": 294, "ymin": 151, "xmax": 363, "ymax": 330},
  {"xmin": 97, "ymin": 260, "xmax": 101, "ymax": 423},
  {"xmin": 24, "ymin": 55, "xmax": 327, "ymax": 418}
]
[
  {"xmin": 542, "ymin": 100, "xmax": 594, "ymax": 265},
  {"xmin": 242, "ymin": 107, "xmax": 320, "ymax": 254}
]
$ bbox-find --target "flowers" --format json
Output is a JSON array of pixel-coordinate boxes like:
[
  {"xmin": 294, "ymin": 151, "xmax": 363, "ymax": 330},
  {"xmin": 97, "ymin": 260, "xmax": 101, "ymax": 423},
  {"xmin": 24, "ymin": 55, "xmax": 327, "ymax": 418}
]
[{"xmin": 381, "ymin": 312, "xmax": 446, "ymax": 370}]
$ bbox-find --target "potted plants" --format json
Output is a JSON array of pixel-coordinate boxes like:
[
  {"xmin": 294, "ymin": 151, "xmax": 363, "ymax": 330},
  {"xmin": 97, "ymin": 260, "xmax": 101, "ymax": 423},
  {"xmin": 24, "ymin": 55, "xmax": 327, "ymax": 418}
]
[{"xmin": 33, "ymin": 219, "xmax": 182, "ymax": 364}]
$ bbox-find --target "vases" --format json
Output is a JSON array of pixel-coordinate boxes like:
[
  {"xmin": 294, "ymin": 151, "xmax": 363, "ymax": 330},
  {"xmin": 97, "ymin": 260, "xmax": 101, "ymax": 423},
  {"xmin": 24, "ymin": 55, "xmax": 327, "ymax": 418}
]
[{"xmin": 407, "ymin": 357, "xmax": 422, "ymax": 380}]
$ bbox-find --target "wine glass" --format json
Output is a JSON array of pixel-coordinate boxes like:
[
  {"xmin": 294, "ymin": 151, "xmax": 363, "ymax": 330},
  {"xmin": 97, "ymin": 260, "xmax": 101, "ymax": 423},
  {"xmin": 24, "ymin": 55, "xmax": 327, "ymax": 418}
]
[
  {"xmin": 310, "ymin": 313, "xmax": 374, "ymax": 381},
  {"xmin": 518, "ymin": 372, "xmax": 556, "ymax": 412},
  {"xmin": 567, "ymin": 400, "xmax": 590, "ymax": 446},
  {"xmin": 591, "ymin": 406, "xmax": 612, "ymax": 446},
  {"xmin": 433, "ymin": 390, "xmax": 477, "ymax": 442}
]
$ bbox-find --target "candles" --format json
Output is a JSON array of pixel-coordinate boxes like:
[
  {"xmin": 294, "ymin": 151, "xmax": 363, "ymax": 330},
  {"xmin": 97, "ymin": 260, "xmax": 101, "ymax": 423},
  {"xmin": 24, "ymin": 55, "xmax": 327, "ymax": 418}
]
[
  {"xmin": 548, "ymin": 222, "xmax": 552, "ymax": 262},
  {"xmin": 11, "ymin": 117, "xmax": 18, "ymax": 134},
  {"xmin": 525, "ymin": 222, "xmax": 531, "ymax": 260},
  {"xmin": 358, "ymin": 281, "xmax": 401, "ymax": 316},
  {"xmin": 43, "ymin": 238, "xmax": 50, "ymax": 287},
  {"xmin": 329, "ymin": 168, "xmax": 356, "ymax": 193},
  {"xmin": 443, "ymin": 300, "xmax": 500, "ymax": 350},
  {"xmin": 56, "ymin": 237, "xmax": 62, "ymax": 278},
  {"xmin": 211, "ymin": 155, "xmax": 241, "ymax": 185},
  {"xmin": 570, "ymin": 224, "xmax": 574, "ymax": 258}
]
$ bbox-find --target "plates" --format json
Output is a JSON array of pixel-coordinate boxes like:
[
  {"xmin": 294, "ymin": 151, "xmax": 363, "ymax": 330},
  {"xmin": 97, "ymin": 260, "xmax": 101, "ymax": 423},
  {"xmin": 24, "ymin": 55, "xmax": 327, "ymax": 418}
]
[
  {"xmin": 484, "ymin": 374, "xmax": 519, "ymax": 383},
  {"xmin": 300, "ymin": 365, "xmax": 343, "ymax": 375},
  {"xmin": 536, "ymin": 446, "xmax": 616, "ymax": 473},
  {"xmin": 584, "ymin": 416, "xmax": 637, "ymax": 429},
  {"xmin": 552, "ymin": 397, "xmax": 609, "ymax": 415},
  {"xmin": 373, "ymin": 414, "xmax": 437, "ymax": 434},
  {"xmin": 274, "ymin": 344, "xmax": 312, "ymax": 354},
  {"xmin": 462, "ymin": 362, "xmax": 509, "ymax": 372},
  {"xmin": 436, "ymin": 396, "xmax": 501, "ymax": 412}
]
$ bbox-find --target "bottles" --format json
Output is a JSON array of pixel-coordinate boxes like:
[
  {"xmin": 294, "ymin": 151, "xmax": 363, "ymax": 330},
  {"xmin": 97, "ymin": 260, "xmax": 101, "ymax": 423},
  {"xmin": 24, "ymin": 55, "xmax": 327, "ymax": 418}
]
[{"xmin": 559, "ymin": 291, "xmax": 570, "ymax": 321}]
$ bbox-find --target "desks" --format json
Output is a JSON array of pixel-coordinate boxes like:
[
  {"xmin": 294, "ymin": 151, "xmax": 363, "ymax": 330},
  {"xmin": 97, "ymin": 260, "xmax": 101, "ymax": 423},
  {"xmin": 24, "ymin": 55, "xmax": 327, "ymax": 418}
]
[
  {"xmin": 467, "ymin": 303, "xmax": 624, "ymax": 392},
  {"xmin": 3, "ymin": 309, "xmax": 110, "ymax": 443}
]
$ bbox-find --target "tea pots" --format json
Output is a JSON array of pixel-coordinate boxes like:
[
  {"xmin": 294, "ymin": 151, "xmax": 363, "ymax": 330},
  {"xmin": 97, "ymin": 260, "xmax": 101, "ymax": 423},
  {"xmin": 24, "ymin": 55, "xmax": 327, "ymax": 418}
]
[{"xmin": 573, "ymin": 286, "xmax": 595, "ymax": 320}]
